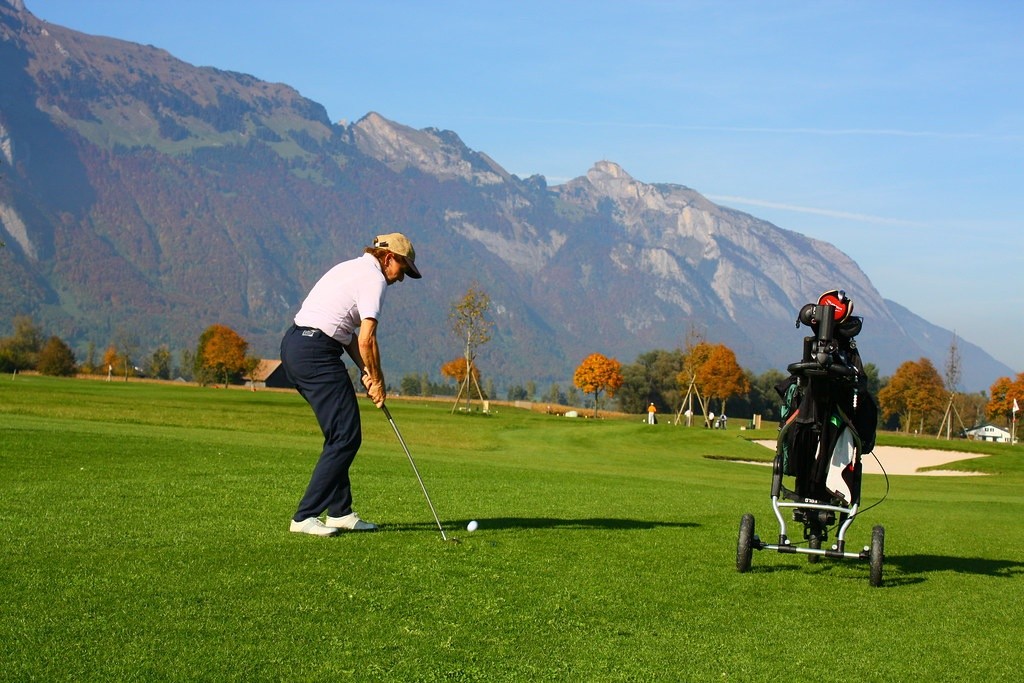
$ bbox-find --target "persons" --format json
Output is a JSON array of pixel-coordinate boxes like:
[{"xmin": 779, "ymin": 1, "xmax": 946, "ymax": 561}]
[
  {"xmin": 647, "ymin": 403, "xmax": 656, "ymax": 424},
  {"xmin": 280, "ymin": 232, "xmax": 416, "ymax": 536},
  {"xmin": 708, "ymin": 411, "xmax": 727, "ymax": 430}
]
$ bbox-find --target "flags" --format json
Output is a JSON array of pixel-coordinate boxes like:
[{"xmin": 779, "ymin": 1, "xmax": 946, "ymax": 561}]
[{"xmin": 1012, "ymin": 397, "xmax": 1019, "ymax": 412}]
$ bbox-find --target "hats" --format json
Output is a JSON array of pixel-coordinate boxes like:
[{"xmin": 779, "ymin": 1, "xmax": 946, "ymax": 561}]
[
  {"xmin": 651, "ymin": 403, "xmax": 654, "ymax": 405},
  {"xmin": 374, "ymin": 233, "xmax": 422, "ymax": 279}
]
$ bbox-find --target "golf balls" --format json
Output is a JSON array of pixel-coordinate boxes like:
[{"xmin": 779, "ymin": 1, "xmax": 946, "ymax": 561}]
[{"xmin": 467, "ymin": 519, "xmax": 479, "ymax": 532}]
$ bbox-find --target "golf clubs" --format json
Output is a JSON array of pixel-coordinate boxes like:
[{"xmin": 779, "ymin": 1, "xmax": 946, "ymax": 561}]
[{"xmin": 360, "ymin": 370, "xmax": 459, "ymax": 543}]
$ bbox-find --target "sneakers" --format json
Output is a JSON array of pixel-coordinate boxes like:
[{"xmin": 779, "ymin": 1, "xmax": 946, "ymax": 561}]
[{"xmin": 289, "ymin": 512, "xmax": 377, "ymax": 537}]
